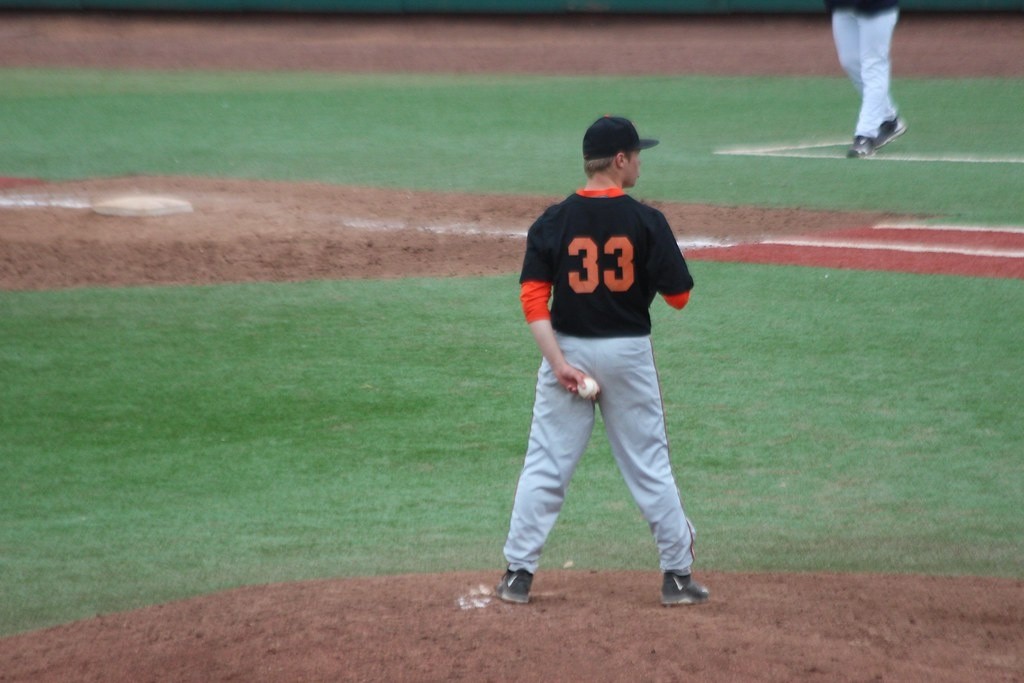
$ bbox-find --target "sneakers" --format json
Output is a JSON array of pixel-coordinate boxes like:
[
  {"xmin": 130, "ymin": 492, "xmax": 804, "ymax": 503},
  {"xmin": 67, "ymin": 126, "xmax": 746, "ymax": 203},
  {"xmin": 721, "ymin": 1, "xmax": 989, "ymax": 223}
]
[
  {"xmin": 872, "ymin": 118, "xmax": 908, "ymax": 148},
  {"xmin": 495, "ymin": 568, "xmax": 532, "ymax": 604},
  {"xmin": 846, "ymin": 136, "xmax": 874, "ymax": 160},
  {"xmin": 660, "ymin": 571, "xmax": 712, "ymax": 604}
]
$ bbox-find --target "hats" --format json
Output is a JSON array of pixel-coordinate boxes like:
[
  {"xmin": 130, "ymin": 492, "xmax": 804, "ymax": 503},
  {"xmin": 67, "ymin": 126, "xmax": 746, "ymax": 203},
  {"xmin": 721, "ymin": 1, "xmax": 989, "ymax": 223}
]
[{"xmin": 583, "ymin": 115, "xmax": 662, "ymax": 161}]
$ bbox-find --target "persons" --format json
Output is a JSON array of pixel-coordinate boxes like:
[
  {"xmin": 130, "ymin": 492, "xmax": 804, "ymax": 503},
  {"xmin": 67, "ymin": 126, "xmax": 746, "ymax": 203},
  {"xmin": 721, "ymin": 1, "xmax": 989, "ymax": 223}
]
[
  {"xmin": 486, "ymin": 113, "xmax": 711, "ymax": 609},
  {"xmin": 828, "ymin": 0, "xmax": 908, "ymax": 163}
]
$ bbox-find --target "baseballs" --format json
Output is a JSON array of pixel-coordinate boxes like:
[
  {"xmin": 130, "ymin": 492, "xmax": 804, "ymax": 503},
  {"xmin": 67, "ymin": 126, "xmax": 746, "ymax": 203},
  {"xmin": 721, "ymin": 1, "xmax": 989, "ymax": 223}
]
[{"xmin": 577, "ymin": 377, "xmax": 597, "ymax": 399}]
